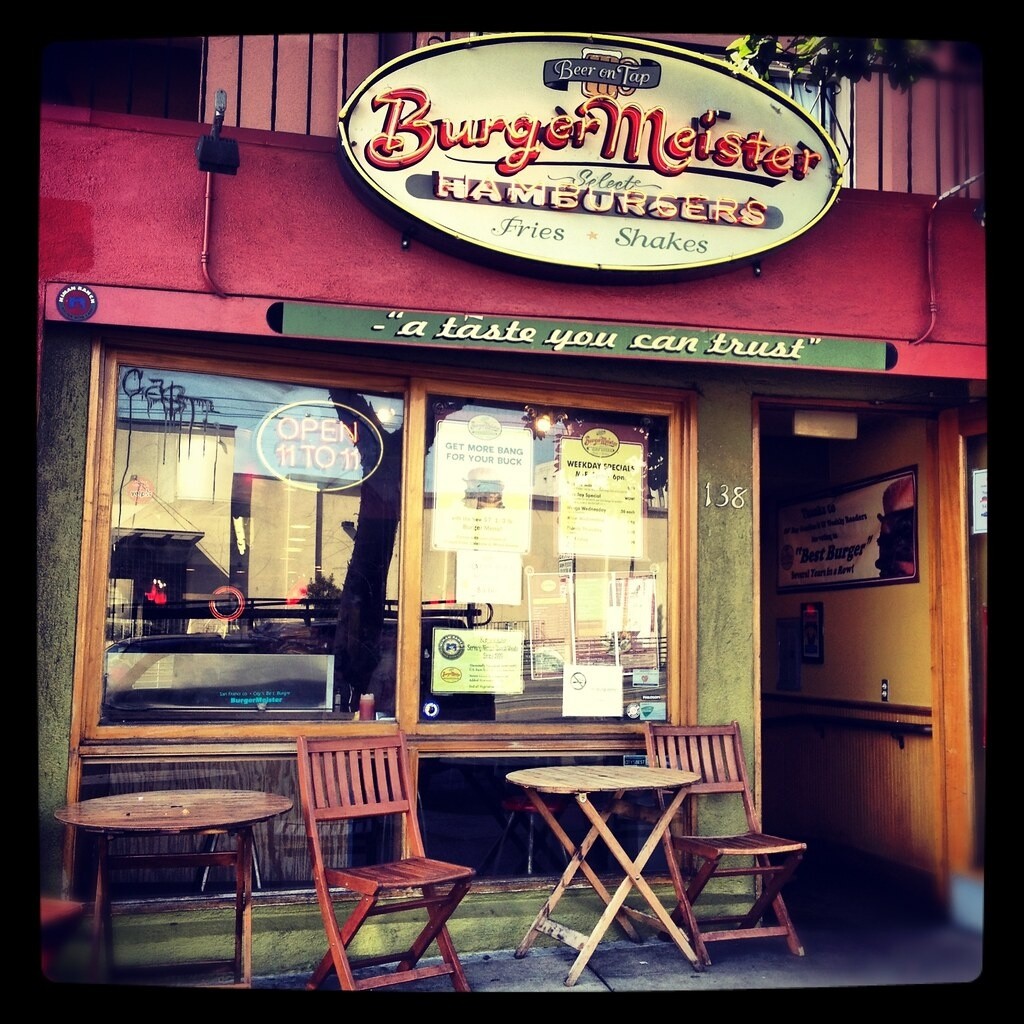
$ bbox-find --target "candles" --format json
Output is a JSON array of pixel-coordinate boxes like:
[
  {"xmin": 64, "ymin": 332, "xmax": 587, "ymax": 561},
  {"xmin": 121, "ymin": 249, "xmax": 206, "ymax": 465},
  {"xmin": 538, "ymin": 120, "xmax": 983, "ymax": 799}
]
[{"xmin": 359, "ymin": 694, "xmax": 375, "ymax": 722}]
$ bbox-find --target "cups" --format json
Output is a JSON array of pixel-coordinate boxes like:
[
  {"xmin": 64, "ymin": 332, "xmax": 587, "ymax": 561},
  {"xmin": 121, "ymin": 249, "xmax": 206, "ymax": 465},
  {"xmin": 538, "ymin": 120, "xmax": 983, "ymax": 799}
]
[{"xmin": 358, "ymin": 700, "xmax": 376, "ymax": 721}]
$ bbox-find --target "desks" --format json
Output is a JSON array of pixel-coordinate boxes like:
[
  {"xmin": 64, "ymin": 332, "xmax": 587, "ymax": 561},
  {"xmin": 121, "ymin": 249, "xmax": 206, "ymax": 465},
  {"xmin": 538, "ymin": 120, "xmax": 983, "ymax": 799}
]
[
  {"xmin": 507, "ymin": 765, "xmax": 701, "ymax": 988},
  {"xmin": 53, "ymin": 787, "xmax": 295, "ymax": 988}
]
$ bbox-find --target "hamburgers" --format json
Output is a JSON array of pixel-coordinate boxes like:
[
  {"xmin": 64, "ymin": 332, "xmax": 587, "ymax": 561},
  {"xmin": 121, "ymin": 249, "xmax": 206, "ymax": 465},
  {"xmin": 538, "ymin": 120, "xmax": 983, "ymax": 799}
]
[{"xmin": 460, "ymin": 467, "xmax": 504, "ymax": 510}]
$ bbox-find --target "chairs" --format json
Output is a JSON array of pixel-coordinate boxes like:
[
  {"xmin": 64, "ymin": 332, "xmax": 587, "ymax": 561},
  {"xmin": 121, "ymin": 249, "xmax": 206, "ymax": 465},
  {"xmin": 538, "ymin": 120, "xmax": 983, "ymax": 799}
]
[
  {"xmin": 295, "ymin": 732, "xmax": 476, "ymax": 992},
  {"xmin": 644, "ymin": 720, "xmax": 807, "ymax": 965}
]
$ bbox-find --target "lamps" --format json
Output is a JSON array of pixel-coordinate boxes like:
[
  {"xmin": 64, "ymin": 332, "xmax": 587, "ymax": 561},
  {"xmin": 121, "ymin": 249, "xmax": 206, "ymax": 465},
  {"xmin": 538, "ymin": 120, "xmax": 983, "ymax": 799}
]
[{"xmin": 194, "ymin": 91, "xmax": 241, "ymax": 175}]
